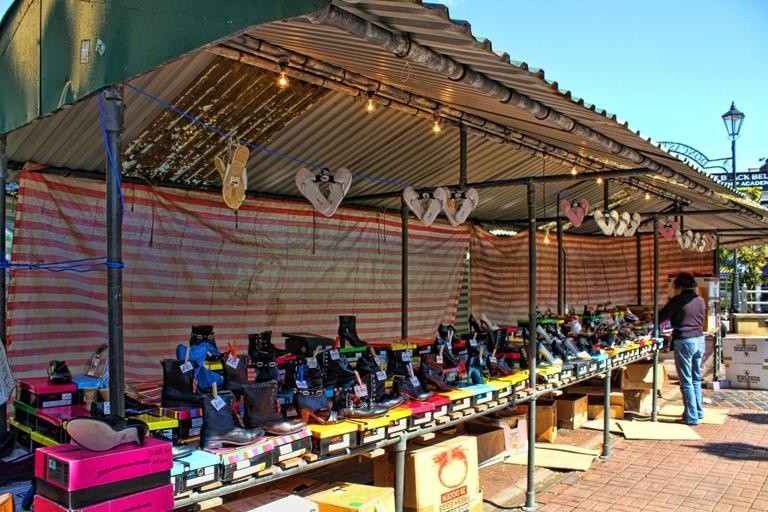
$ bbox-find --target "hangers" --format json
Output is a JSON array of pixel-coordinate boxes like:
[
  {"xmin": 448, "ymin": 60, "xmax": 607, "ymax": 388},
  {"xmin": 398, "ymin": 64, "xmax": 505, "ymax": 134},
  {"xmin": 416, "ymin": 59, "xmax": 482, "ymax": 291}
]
[
  {"xmin": 310, "ymin": 167, "xmax": 337, "ymax": 184},
  {"xmin": 599, "ymin": 207, "xmax": 714, "ymax": 242},
  {"xmin": 448, "ymin": 186, "xmax": 469, "ymax": 201},
  {"xmin": 570, "ymin": 199, "xmax": 581, "ymax": 208},
  {"xmin": 417, "ymin": 186, "xmax": 437, "ymax": 201}
]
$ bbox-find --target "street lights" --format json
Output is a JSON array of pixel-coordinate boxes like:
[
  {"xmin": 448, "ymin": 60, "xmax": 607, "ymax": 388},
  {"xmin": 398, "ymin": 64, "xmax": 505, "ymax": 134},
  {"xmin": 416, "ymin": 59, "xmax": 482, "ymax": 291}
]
[{"xmin": 720, "ymin": 97, "xmax": 746, "ymax": 314}]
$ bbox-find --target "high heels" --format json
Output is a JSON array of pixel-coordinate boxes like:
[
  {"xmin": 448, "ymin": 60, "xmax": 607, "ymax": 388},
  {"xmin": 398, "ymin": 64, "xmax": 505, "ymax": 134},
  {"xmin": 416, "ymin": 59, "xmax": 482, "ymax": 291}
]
[
  {"xmin": 65, "ymin": 414, "xmax": 149, "ymax": 450},
  {"xmin": 160, "ymin": 301, "xmax": 651, "ymax": 449}
]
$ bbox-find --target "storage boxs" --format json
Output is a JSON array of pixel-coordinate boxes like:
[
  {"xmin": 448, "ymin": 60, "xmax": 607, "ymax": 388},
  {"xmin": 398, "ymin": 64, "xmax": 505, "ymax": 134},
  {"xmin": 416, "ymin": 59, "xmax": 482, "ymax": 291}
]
[
  {"xmin": 6, "ymin": 376, "xmax": 107, "ymax": 512},
  {"xmin": 339, "ymin": 331, "xmax": 532, "ymax": 441},
  {"xmin": 34, "ymin": 439, "xmax": 171, "ymax": 506},
  {"xmin": 725, "ymin": 364, "xmax": 768, "ymax": 390},
  {"xmin": 33, "ymin": 484, "xmax": 173, "ymax": 512},
  {"xmin": 211, "ymin": 490, "xmax": 318, "ymax": 512},
  {"xmin": 305, "ymin": 482, "xmax": 397, "ymax": 512},
  {"xmin": 479, "ymin": 422, "xmax": 526, "ymax": 464},
  {"xmin": 449, "ymin": 490, "xmax": 486, "ymax": 512},
  {"xmin": 721, "ymin": 336, "xmax": 768, "ymax": 364},
  {"xmin": 375, "ymin": 434, "xmax": 479, "ymax": 510},
  {"xmin": 536, "ymin": 305, "xmax": 668, "ymax": 458},
  {"xmin": 135, "ymin": 352, "xmax": 379, "ymax": 497}
]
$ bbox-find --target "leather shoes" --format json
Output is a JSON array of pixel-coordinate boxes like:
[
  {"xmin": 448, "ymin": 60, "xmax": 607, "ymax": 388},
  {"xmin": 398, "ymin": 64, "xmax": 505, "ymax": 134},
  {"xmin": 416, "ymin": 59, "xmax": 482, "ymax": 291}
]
[{"xmin": 47, "ymin": 358, "xmax": 73, "ymax": 384}]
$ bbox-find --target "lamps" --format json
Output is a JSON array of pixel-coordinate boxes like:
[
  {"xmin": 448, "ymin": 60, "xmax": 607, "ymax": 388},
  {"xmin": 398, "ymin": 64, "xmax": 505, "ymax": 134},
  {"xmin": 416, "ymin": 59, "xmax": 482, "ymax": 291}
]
[
  {"xmin": 570, "ymin": 163, "xmax": 577, "ymax": 174},
  {"xmin": 365, "ymin": 91, "xmax": 376, "ymax": 114},
  {"xmin": 537, "ymin": 158, "xmax": 557, "ymax": 245},
  {"xmin": 645, "ymin": 190, "xmax": 651, "ymax": 201},
  {"xmin": 275, "ymin": 55, "xmax": 290, "ymax": 88},
  {"xmin": 430, "ymin": 105, "xmax": 442, "ymax": 133}
]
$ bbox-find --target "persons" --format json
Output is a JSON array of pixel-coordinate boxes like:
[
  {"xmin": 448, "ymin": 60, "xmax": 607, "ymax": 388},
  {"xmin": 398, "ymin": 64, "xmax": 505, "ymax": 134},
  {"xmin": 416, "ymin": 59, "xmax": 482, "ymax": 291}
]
[{"xmin": 659, "ymin": 272, "xmax": 705, "ymax": 425}]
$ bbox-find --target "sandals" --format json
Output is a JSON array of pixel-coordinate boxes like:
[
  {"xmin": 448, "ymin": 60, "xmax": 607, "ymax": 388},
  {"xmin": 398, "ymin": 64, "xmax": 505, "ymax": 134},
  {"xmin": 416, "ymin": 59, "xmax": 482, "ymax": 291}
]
[
  {"xmin": 214, "ymin": 145, "xmax": 250, "ymax": 209},
  {"xmin": 559, "ymin": 198, "xmax": 717, "ymax": 253},
  {"xmin": 295, "ymin": 166, "xmax": 353, "ymax": 216},
  {"xmin": 402, "ymin": 185, "xmax": 480, "ymax": 228}
]
